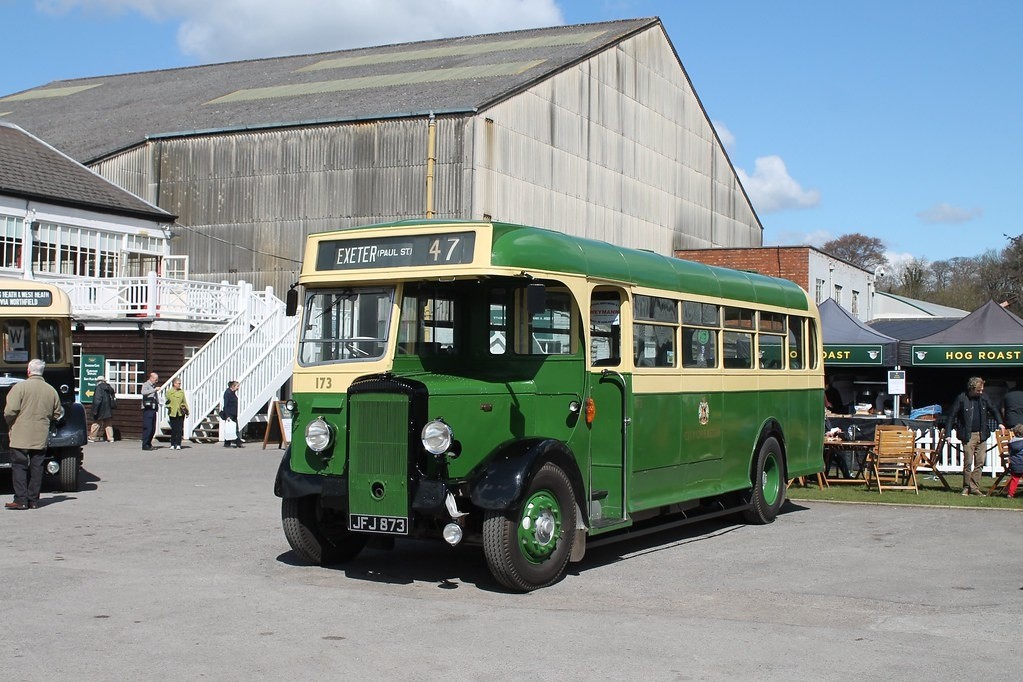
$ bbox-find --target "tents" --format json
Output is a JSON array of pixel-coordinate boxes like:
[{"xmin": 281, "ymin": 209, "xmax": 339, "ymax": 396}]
[{"xmin": 735, "ymin": 296, "xmax": 1023, "ymax": 419}]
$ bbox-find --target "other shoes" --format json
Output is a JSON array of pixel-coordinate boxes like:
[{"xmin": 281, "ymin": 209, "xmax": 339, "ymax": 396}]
[
  {"xmin": 4, "ymin": 502, "xmax": 28, "ymax": 509},
  {"xmin": 1007, "ymin": 494, "xmax": 1014, "ymax": 498},
  {"xmin": 175, "ymin": 445, "xmax": 181, "ymax": 450},
  {"xmin": 28, "ymin": 503, "xmax": 38, "ymax": 509},
  {"xmin": 236, "ymin": 444, "xmax": 246, "ymax": 448},
  {"xmin": 88, "ymin": 437, "xmax": 94, "ymax": 442},
  {"xmin": 142, "ymin": 444, "xmax": 158, "ymax": 450},
  {"xmin": 224, "ymin": 442, "xmax": 236, "ymax": 447},
  {"xmin": 105, "ymin": 440, "xmax": 115, "ymax": 443},
  {"xmin": 169, "ymin": 446, "xmax": 175, "ymax": 450}
]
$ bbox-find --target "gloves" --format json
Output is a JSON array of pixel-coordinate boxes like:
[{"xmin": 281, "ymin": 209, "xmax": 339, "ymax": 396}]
[
  {"xmin": 54, "ymin": 418, "xmax": 66, "ymax": 429},
  {"xmin": 185, "ymin": 410, "xmax": 189, "ymax": 414},
  {"xmin": 93, "ymin": 415, "xmax": 98, "ymax": 420},
  {"xmin": 165, "ymin": 399, "xmax": 171, "ymax": 404}
]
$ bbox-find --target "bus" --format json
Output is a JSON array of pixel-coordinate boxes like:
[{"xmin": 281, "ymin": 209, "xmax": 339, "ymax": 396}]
[
  {"xmin": 274, "ymin": 218, "xmax": 826, "ymax": 591},
  {"xmin": 0, "ymin": 279, "xmax": 88, "ymax": 489}
]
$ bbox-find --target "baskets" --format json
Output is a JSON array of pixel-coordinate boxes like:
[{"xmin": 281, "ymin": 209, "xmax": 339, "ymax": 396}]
[{"xmin": 910, "ymin": 405, "xmax": 935, "ymax": 420}]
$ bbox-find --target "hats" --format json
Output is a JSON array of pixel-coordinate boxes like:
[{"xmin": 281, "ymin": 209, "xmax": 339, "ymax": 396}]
[{"xmin": 96, "ymin": 376, "xmax": 105, "ymax": 381}]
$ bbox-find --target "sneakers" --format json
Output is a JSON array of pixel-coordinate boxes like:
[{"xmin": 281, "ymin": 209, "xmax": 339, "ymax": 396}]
[
  {"xmin": 969, "ymin": 490, "xmax": 988, "ymax": 497},
  {"xmin": 961, "ymin": 486, "xmax": 969, "ymax": 496}
]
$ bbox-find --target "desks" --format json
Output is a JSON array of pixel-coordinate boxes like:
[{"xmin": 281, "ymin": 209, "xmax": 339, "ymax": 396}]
[{"xmin": 823, "ymin": 440, "xmax": 877, "ymax": 490}]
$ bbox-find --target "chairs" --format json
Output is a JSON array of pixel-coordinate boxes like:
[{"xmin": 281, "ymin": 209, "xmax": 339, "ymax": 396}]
[
  {"xmin": 864, "ymin": 423, "xmax": 920, "ymax": 494},
  {"xmin": 986, "ymin": 429, "xmax": 1015, "ymax": 495},
  {"xmin": 906, "ymin": 428, "xmax": 952, "ymax": 490}
]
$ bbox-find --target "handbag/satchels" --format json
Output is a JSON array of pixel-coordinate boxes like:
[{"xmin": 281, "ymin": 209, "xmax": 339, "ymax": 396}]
[
  {"xmin": 110, "ymin": 393, "xmax": 118, "ymax": 409},
  {"xmin": 224, "ymin": 419, "xmax": 238, "ymax": 441},
  {"xmin": 180, "ymin": 403, "xmax": 186, "ymax": 415},
  {"xmin": 219, "ymin": 410, "xmax": 226, "ymax": 420},
  {"xmin": 143, "ymin": 392, "xmax": 157, "ymax": 410}
]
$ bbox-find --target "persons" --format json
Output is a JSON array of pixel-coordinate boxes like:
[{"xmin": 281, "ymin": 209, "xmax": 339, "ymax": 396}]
[
  {"xmin": 220, "ymin": 380, "xmax": 246, "ymax": 448},
  {"xmin": 87, "ymin": 376, "xmax": 118, "ymax": 443},
  {"xmin": 141, "ymin": 373, "xmax": 161, "ymax": 450},
  {"xmin": 165, "ymin": 378, "xmax": 189, "ymax": 450},
  {"xmin": 4, "ymin": 359, "xmax": 61, "ymax": 510},
  {"xmin": 824, "ymin": 417, "xmax": 853, "ymax": 479},
  {"xmin": 946, "ymin": 377, "xmax": 1006, "ymax": 496},
  {"xmin": 1006, "ymin": 424, "xmax": 1023, "ymax": 498},
  {"xmin": 1001, "ymin": 391, "xmax": 1023, "ymax": 429}
]
